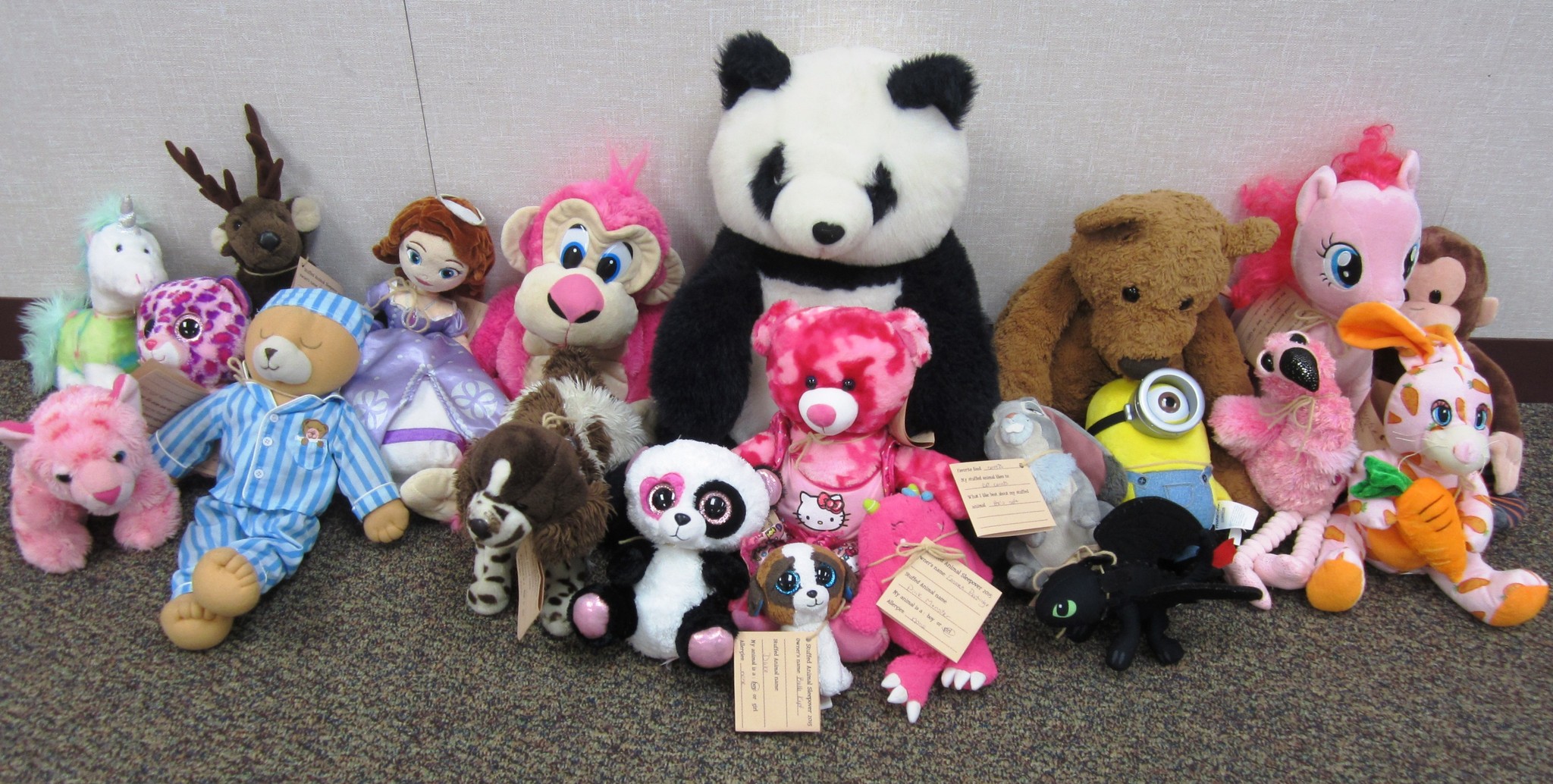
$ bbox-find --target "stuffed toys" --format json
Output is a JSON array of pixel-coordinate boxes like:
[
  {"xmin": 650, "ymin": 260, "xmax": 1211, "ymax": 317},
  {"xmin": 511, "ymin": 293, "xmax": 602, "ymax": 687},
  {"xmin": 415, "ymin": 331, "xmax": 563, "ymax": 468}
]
[{"xmin": 0, "ymin": 28, "xmax": 1552, "ymax": 724}]
